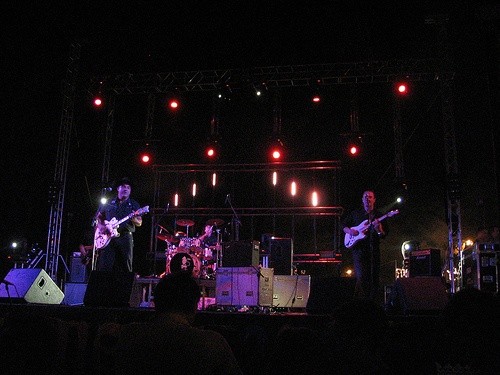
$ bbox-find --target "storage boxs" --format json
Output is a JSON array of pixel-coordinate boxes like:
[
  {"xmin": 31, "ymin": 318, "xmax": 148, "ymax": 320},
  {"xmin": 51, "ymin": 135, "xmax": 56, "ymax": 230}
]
[{"xmin": 462, "ymin": 242, "xmax": 500, "ymax": 294}]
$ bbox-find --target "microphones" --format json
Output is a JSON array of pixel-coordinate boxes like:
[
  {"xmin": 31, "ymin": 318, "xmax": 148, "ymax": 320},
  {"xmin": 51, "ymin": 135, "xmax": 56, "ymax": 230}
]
[
  {"xmin": 225, "ymin": 194, "xmax": 229, "ymax": 203},
  {"xmin": 167, "ymin": 203, "xmax": 169, "ymax": 211},
  {"xmin": 0, "ymin": 279, "xmax": 13, "ymax": 285}
]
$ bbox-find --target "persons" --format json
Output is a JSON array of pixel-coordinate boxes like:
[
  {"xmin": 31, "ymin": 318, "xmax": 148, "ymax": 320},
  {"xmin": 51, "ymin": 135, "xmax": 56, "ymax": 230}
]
[
  {"xmin": 96, "ymin": 179, "xmax": 142, "ymax": 273},
  {"xmin": 200, "ymin": 225, "xmax": 221, "ymax": 252},
  {"xmin": 80, "ymin": 231, "xmax": 93, "ymax": 282},
  {"xmin": 0, "ymin": 269, "xmax": 500, "ymax": 375},
  {"xmin": 340, "ymin": 191, "xmax": 389, "ymax": 303}
]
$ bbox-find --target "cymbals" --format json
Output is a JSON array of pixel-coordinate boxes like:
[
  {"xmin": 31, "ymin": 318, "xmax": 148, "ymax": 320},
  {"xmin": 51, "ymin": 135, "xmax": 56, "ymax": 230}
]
[
  {"xmin": 158, "ymin": 223, "xmax": 169, "ymax": 233},
  {"xmin": 157, "ymin": 235, "xmax": 179, "ymax": 242},
  {"xmin": 173, "ymin": 230, "xmax": 186, "ymax": 236},
  {"xmin": 176, "ymin": 219, "xmax": 195, "ymax": 226},
  {"xmin": 207, "ymin": 219, "xmax": 225, "ymax": 225}
]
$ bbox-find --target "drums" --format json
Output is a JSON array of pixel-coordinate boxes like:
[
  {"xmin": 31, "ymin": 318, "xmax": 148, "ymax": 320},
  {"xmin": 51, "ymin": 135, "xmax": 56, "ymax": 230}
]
[
  {"xmin": 168, "ymin": 246, "xmax": 189, "ymax": 260},
  {"xmin": 195, "ymin": 248, "xmax": 213, "ymax": 260},
  {"xmin": 169, "ymin": 255, "xmax": 201, "ymax": 278},
  {"xmin": 190, "ymin": 237, "xmax": 202, "ymax": 252},
  {"xmin": 180, "ymin": 238, "xmax": 191, "ymax": 250}
]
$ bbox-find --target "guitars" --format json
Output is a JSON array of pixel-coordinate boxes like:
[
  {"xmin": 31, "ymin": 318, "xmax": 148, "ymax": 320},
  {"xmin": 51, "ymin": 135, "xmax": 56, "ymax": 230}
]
[
  {"xmin": 344, "ymin": 210, "xmax": 399, "ymax": 248},
  {"xmin": 94, "ymin": 205, "xmax": 149, "ymax": 249}
]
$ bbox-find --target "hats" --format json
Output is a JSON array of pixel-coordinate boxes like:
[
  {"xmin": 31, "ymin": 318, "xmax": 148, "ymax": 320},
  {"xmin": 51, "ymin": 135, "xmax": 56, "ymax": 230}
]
[{"xmin": 117, "ymin": 178, "xmax": 131, "ymax": 187}]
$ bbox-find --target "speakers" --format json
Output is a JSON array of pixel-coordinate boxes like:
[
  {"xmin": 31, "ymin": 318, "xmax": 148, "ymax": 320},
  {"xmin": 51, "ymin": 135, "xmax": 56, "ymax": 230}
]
[
  {"xmin": 84, "ymin": 270, "xmax": 143, "ymax": 309},
  {"xmin": 394, "ymin": 248, "xmax": 448, "ymax": 315},
  {"xmin": 215, "ymin": 238, "xmax": 361, "ymax": 315},
  {"xmin": 0, "ymin": 258, "xmax": 91, "ymax": 306}
]
